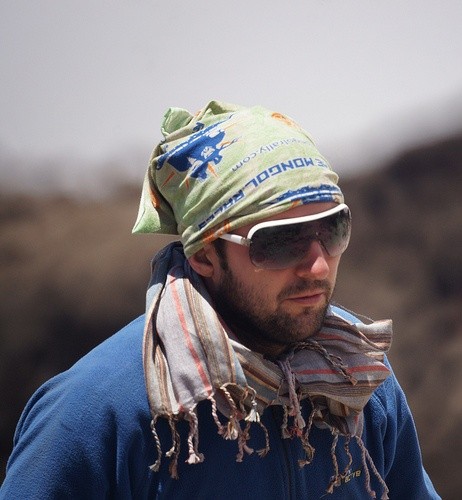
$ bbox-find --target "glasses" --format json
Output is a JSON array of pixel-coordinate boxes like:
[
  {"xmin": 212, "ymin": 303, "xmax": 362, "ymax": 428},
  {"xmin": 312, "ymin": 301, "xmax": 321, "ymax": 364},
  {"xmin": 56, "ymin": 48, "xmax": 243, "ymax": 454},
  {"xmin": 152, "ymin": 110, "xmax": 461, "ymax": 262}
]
[{"xmin": 219, "ymin": 204, "xmax": 352, "ymax": 270}]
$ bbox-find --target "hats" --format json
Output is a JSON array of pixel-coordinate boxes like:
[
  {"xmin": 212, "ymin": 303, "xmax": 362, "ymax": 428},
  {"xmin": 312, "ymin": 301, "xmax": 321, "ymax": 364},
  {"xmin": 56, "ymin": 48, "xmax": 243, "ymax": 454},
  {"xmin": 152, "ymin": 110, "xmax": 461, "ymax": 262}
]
[{"xmin": 131, "ymin": 100, "xmax": 344, "ymax": 260}]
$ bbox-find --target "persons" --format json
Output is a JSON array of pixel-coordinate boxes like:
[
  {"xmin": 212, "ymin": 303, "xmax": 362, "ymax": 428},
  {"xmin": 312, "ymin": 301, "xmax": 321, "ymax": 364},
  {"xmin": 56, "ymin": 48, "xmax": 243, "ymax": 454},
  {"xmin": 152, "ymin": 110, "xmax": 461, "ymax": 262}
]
[{"xmin": 0, "ymin": 101, "xmax": 441, "ymax": 500}]
